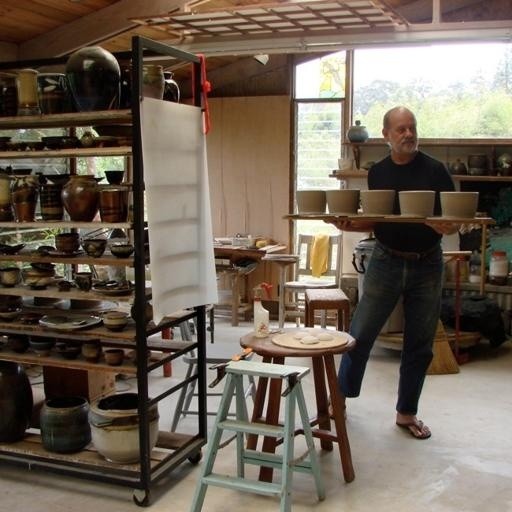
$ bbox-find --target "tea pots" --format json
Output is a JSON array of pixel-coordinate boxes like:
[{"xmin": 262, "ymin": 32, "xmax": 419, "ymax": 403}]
[{"xmin": 446, "ymin": 158, "xmax": 468, "ymax": 176}]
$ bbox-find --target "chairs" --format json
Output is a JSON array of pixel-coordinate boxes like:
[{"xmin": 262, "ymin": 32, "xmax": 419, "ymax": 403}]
[{"xmin": 278, "ymin": 233, "xmax": 343, "ymax": 329}]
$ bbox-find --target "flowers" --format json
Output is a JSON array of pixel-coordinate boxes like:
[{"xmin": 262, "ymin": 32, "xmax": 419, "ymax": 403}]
[{"xmin": 252, "ymin": 282, "xmax": 272, "ymax": 300}]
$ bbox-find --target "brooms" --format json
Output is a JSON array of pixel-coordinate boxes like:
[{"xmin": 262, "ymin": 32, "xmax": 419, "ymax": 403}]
[{"xmin": 425, "ymin": 318, "xmax": 460, "ymax": 375}]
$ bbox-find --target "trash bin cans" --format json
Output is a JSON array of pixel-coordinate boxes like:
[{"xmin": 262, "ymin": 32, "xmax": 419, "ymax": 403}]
[{"xmin": 352, "ymin": 239, "xmax": 405, "ymax": 335}]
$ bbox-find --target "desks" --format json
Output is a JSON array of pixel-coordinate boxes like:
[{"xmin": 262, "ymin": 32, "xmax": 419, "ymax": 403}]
[
  {"xmin": 240, "ymin": 326, "xmax": 355, "ymax": 484},
  {"xmin": 212, "ymin": 240, "xmax": 300, "ymax": 326}
]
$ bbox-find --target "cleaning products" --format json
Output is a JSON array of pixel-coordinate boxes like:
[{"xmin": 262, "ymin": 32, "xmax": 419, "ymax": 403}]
[{"xmin": 253, "ymin": 282, "xmax": 273, "ymax": 338}]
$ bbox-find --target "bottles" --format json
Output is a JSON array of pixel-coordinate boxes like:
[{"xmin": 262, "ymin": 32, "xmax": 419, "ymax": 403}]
[
  {"xmin": 489, "ymin": 250, "xmax": 509, "ymax": 283},
  {"xmin": 469, "ymin": 260, "xmax": 484, "ymax": 283},
  {"xmin": 253, "ymin": 295, "xmax": 270, "ymax": 337}
]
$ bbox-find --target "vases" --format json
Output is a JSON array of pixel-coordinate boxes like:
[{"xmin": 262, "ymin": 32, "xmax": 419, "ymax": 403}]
[
  {"xmin": 252, "ymin": 299, "xmax": 269, "ymax": 338},
  {"xmin": 0, "ymin": 165, "xmax": 129, "ymax": 223}
]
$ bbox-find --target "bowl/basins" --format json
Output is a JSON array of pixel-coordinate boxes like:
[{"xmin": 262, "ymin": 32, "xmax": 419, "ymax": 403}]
[
  {"xmin": 295, "ymin": 190, "xmax": 327, "ymax": 214},
  {"xmin": 80, "ymin": 238, "xmax": 108, "ymax": 256},
  {"xmin": 12, "ymin": 167, "xmax": 33, "ymax": 176},
  {"xmin": 326, "ymin": 188, "xmax": 360, "ymax": 214},
  {"xmin": 91, "ymin": 123, "xmax": 134, "ymax": 137},
  {"xmin": 42, "ymin": 172, "xmax": 76, "ymax": 185},
  {"xmin": 38, "ymin": 135, "xmax": 78, "ymax": 149},
  {"xmin": 54, "ymin": 231, "xmax": 80, "ymax": 253},
  {"xmin": 73, "ymin": 271, "xmax": 95, "ymax": 291},
  {"xmin": 399, "ymin": 190, "xmax": 436, "ymax": 218},
  {"xmin": 0, "ymin": 334, "xmax": 125, "ymax": 366},
  {"xmin": 338, "ymin": 158, "xmax": 354, "ymax": 170},
  {"xmin": 361, "ymin": 189, "xmax": 397, "ymax": 214},
  {"xmin": 104, "ymin": 170, "xmax": 125, "ymax": 184},
  {"xmin": 439, "ymin": 190, "xmax": 479, "ymax": 218},
  {"xmin": 6, "ymin": 140, "xmax": 44, "ymax": 151},
  {"xmin": 103, "ymin": 312, "xmax": 128, "ymax": 333},
  {"xmin": 110, "ymin": 244, "xmax": 134, "ymax": 258}
]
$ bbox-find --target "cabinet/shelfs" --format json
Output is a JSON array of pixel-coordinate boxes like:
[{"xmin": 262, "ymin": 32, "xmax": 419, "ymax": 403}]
[
  {"xmin": 328, "ymin": 137, "xmax": 512, "ymax": 341},
  {"xmin": 0, "ymin": 34, "xmax": 208, "ymax": 508}
]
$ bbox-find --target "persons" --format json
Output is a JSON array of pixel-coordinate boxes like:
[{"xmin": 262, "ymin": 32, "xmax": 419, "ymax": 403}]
[{"xmin": 328, "ymin": 106, "xmax": 462, "ymax": 440}]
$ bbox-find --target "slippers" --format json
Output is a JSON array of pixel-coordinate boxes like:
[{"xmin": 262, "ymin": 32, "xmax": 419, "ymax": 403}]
[{"xmin": 396, "ymin": 417, "xmax": 431, "ymax": 440}]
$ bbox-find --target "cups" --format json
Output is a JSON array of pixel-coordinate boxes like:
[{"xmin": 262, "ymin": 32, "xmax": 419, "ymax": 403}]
[{"xmin": 1, "ymin": 267, "xmax": 20, "ymax": 289}]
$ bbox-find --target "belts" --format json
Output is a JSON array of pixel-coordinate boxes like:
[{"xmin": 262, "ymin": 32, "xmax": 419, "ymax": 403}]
[{"xmin": 376, "ymin": 241, "xmax": 439, "ymax": 259}]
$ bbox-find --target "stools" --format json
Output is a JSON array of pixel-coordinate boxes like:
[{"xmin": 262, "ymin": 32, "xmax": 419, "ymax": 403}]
[
  {"xmin": 304, "ymin": 288, "xmax": 350, "ymax": 334},
  {"xmin": 189, "ymin": 360, "xmax": 326, "ymax": 512},
  {"xmin": 170, "ymin": 345, "xmax": 256, "ymax": 441}
]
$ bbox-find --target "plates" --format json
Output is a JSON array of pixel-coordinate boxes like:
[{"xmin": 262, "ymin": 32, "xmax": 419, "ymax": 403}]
[
  {"xmin": 212, "ymin": 236, "xmax": 232, "ymax": 248},
  {"xmin": 1, "ymin": 297, "xmax": 120, "ymax": 331},
  {"xmin": 0, "ymin": 243, "xmax": 27, "ymax": 255},
  {"xmin": 89, "ymin": 285, "xmax": 138, "ymax": 296},
  {"xmin": 48, "ymin": 249, "xmax": 81, "ymax": 257}
]
